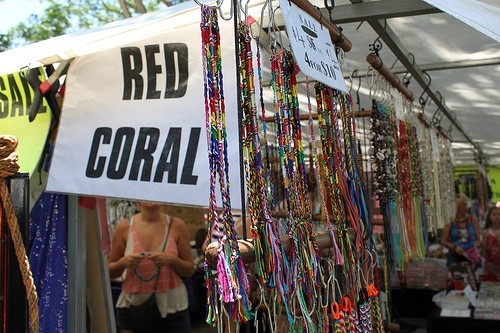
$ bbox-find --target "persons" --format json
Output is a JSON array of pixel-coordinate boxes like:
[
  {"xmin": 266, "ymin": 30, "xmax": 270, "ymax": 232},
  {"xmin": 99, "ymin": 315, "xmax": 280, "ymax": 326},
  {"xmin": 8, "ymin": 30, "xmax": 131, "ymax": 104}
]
[
  {"xmin": 482, "ymin": 205, "xmax": 500, "ymax": 279},
  {"xmin": 440, "ymin": 195, "xmax": 483, "ymax": 270},
  {"xmin": 106, "ymin": 200, "xmax": 197, "ymax": 333}
]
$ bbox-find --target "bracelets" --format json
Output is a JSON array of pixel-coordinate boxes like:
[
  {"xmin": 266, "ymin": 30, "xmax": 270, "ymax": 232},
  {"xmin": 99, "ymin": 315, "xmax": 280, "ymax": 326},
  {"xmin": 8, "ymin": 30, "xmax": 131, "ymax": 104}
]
[{"xmin": 451, "ymin": 245, "xmax": 457, "ymax": 253}]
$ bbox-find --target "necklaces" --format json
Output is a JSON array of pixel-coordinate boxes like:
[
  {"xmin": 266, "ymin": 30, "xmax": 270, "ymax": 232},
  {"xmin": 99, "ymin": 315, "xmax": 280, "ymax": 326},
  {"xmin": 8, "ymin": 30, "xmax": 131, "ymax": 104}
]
[
  {"xmin": 455, "ymin": 215, "xmax": 468, "ymax": 224},
  {"xmin": 134, "ymin": 251, "xmax": 160, "ymax": 282}
]
